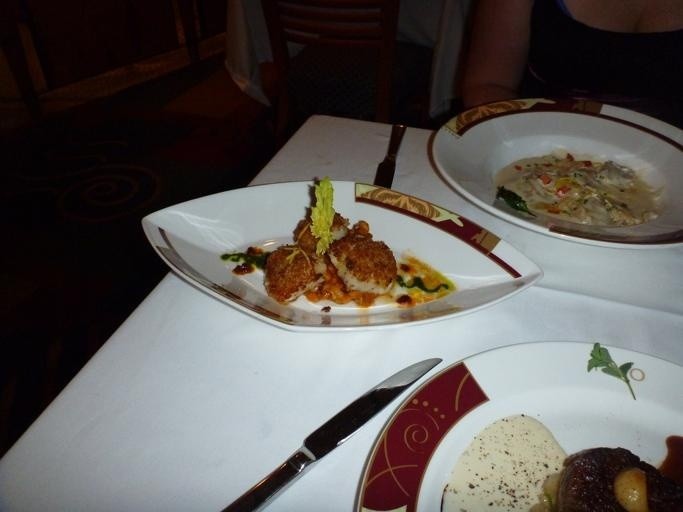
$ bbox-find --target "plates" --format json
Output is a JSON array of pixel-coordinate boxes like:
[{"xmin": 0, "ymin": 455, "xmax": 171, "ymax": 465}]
[
  {"xmin": 141, "ymin": 180, "xmax": 543, "ymax": 331},
  {"xmin": 429, "ymin": 95, "xmax": 681, "ymax": 245},
  {"xmin": 357, "ymin": 341, "xmax": 682, "ymax": 512}
]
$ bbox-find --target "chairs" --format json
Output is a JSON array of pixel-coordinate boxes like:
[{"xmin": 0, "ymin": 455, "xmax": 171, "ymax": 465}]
[{"xmin": 258, "ymin": 0, "xmax": 413, "ymax": 155}]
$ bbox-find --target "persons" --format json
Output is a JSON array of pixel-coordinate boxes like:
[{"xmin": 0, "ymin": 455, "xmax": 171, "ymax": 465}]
[{"xmin": 462, "ymin": 0, "xmax": 683, "ymax": 129}]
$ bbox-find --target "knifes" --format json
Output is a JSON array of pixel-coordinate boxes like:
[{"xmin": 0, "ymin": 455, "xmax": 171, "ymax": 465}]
[
  {"xmin": 213, "ymin": 356, "xmax": 446, "ymax": 511},
  {"xmin": 372, "ymin": 117, "xmax": 412, "ymax": 189}
]
